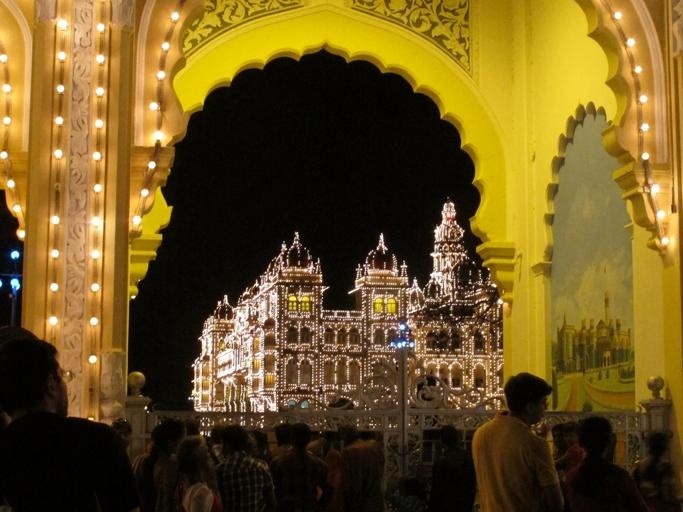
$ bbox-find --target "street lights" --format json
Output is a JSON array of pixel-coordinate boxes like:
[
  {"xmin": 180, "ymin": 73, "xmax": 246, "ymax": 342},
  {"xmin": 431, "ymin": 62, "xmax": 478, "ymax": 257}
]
[{"xmin": 9, "ymin": 250, "xmax": 21, "ymax": 327}]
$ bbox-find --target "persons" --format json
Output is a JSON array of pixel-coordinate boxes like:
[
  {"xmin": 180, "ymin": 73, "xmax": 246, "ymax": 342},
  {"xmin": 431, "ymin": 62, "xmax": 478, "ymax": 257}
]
[
  {"xmin": 473, "ymin": 373, "xmax": 565, "ymax": 512},
  {"xmin": 112, "ymin": 418, "xmax": 385, "ymax": 512},
  {"xmin": 551, "ymin": 417, "xmax": 682, "ymax": 511},
  {"xmin": 426, "ymin": 425, "xmax": 476, "ymax": 512},
  {"xmin": 0, "ymin": 325, "xmax": 140, "ymax": 512}
]
